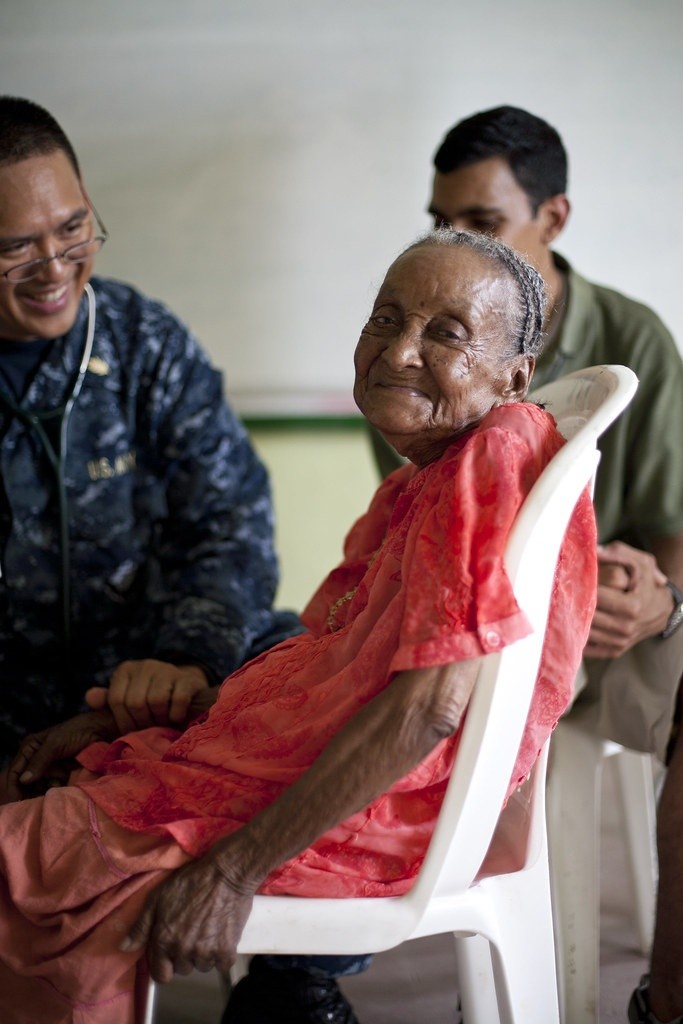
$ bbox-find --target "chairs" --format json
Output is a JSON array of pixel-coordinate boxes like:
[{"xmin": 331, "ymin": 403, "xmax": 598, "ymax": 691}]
[{"xmin": 134, "ymin": 364, "xmax": 664, "ymax": 1024}]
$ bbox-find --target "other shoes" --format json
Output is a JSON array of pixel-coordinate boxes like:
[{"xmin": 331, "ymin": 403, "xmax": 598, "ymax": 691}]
[{"xmin": 218, "ymin": 970, "xmax": 359, "ymax": 1024}]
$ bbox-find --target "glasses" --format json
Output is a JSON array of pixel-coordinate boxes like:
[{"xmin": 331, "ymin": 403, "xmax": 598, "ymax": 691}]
[{"xmin": 0, "ymin": 186, "xmax": 110, "ymax": 285}]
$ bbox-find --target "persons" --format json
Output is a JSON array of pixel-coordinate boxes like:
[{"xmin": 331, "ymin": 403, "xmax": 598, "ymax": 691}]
[
  {"xmin": 367, "ymin": 106, "xmax": 683, "ymax": 1024},
  {"xmin": 0, "ymin": 96, "xmax": 373, "ymax": 1024},
  {"xmin": 0, "ymin": 227, "xmax": 597, "ymax": 1023}
]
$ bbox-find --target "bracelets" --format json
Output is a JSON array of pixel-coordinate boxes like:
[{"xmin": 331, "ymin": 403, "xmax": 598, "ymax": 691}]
[{"xmin": 662, "ymin": 581, "xmax": 683, "ymax": 641}]
